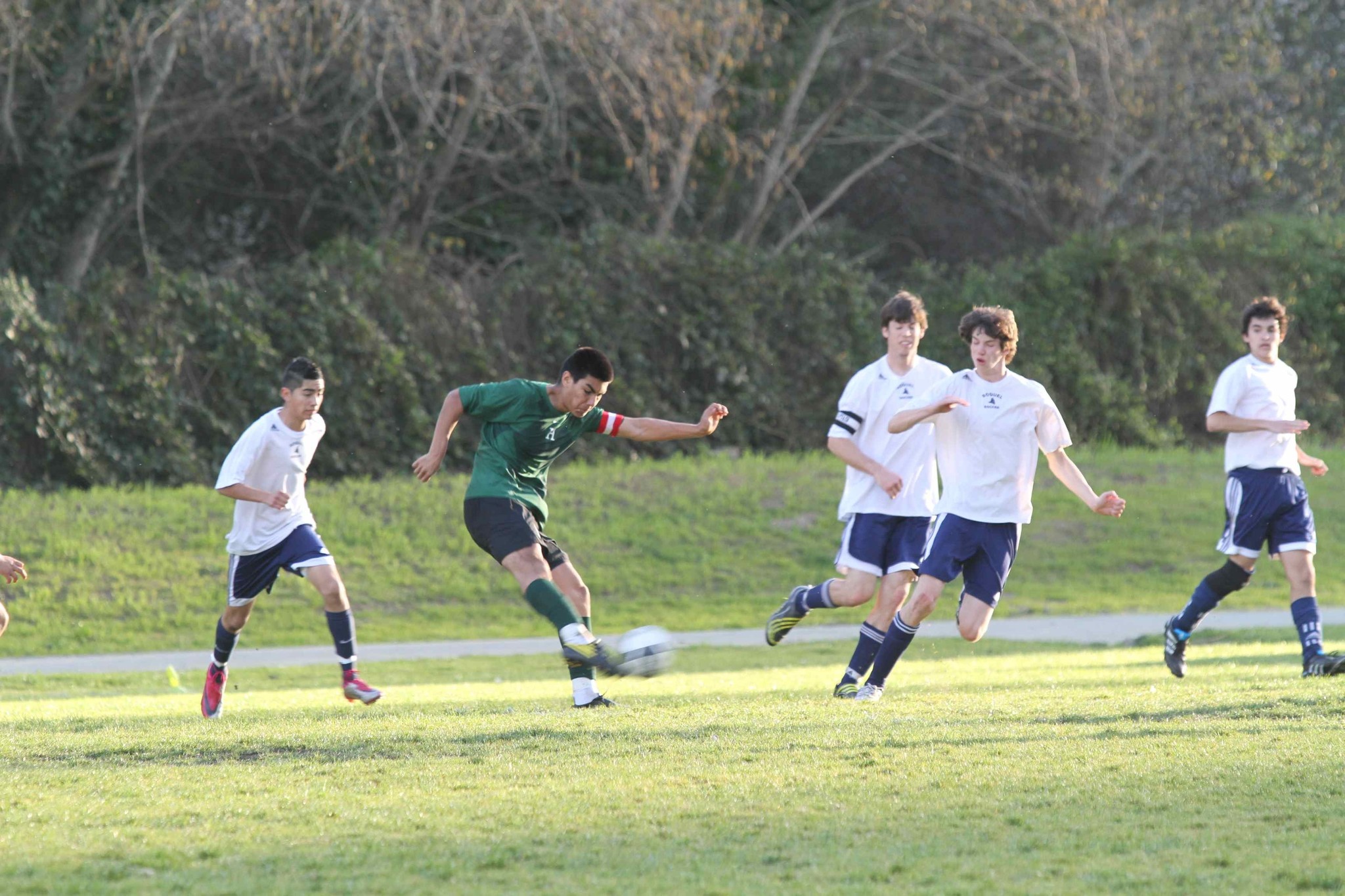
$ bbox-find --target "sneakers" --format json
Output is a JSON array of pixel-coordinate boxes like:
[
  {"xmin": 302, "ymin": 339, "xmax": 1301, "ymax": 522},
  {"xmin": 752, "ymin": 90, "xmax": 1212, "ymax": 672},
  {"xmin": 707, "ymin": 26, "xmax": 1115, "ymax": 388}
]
[
  {"xmin": 832, "ymin": 682, "xmax": 859, "ymax": 699},
  {"xmin": 200, "ymin": 662, "xmax": 228, "ymax": 720},
  {"xmin": 853, "ymin": 685, "xmax": 885, "ymax": 702},
  {"xmin": 343, "ymin": 679, "xmax": 382, "ymax": 705},
  {"xmin": 562, "ymin": 638, "xmax": 629, "ymax": 677},
  {"xmin": 571, "ymin": 695, "xmax": 626, "ymax": 709},
  {"xmin": 1163, "ymin": 615, "xmax": 1192, "ymax": 678},
  {"xmin": 1301, "ymin": 651, "xmax": 1345, "ymax": 678},
  {"xmin": 765, "ymin": 585, "xmax": 812, "ymax": 646}
]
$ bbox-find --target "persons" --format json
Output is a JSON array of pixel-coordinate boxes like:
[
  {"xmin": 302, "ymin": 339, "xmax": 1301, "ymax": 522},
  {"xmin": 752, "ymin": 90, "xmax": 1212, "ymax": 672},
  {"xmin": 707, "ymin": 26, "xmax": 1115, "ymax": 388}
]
[
  {"xmin": 1165, "ymin": 296, "xmax": 1345, "ymax": 678},
  {"xmin": 201, "ymin": 357, "xmax": 382, "ymax": 719},
  {"xmin": 1, "ymin": 554, "xmax": 24, "ymax": 629},
  {"xmin": 851, "ymin": 301, "xmax": 1125, "ymax": 704},
  {"xmin": 410, "ymin": 346, "xmax": 728, "ymax": 708},
  {"xmin": 765, "ymin": 292, "xmax": 956, "ymax": 702}
]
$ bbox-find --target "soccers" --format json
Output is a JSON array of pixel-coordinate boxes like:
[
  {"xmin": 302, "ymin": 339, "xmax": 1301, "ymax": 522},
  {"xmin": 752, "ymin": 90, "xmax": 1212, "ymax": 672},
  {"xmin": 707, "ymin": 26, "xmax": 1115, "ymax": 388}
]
[{"xmin": 611, "ymin": 625, "xmax": 678, "ymax": 677}]
